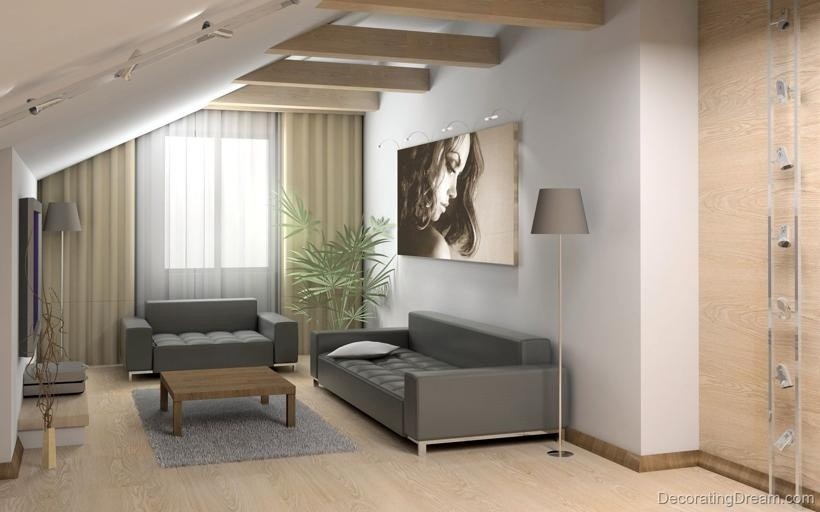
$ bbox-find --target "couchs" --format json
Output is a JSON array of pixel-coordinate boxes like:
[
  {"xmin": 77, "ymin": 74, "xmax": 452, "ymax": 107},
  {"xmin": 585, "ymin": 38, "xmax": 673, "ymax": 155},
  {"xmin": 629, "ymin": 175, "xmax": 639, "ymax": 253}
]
[
  {"xmin": 308, "ymin": 308, "xmax": 574, "ymax": 460},
  {"xmin": 120, "ymin": 297, "xmax": 300, "ymax": 385}
]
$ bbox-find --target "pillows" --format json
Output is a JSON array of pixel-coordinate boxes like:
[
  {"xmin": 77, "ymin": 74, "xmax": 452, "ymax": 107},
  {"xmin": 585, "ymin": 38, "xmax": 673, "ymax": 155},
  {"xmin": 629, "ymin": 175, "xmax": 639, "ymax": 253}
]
[{"xmin": 324, "ymin": 338, "xmax": 402, "ymax": 361}]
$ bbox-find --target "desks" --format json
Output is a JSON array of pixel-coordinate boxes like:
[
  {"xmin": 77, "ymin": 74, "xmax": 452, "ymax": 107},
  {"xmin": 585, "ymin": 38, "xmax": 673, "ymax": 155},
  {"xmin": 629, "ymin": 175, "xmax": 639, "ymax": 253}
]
[{"xmin": 16, "ymin": 387, "xmax": 91, "ymax": 451}]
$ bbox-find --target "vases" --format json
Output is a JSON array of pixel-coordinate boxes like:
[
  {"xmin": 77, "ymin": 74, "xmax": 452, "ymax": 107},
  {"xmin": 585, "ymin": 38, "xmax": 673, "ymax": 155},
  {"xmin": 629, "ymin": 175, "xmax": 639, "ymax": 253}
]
[{"xmin": 41, "ymin": 426, "xmax": 58, "ymax": 470}]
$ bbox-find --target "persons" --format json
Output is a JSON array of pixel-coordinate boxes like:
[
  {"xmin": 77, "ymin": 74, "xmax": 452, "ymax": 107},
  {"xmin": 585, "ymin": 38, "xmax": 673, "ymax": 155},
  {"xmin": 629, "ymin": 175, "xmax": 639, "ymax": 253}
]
[{"xmin": 396, "ymin": 131, "xmax": 485, "ymax": 259}]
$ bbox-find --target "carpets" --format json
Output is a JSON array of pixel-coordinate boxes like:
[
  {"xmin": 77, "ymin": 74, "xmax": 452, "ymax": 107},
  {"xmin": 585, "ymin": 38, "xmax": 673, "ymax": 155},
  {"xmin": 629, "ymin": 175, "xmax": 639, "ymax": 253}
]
[{"xmin": 130, "ymin": 387, "xmax": 362, "ymax": 471}]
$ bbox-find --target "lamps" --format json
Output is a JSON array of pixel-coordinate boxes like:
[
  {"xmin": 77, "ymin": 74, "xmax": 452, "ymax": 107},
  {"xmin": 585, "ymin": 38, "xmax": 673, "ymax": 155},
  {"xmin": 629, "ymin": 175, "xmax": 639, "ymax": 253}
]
[
  {"xmin": 528, "ymin": 186, "xmax": 591, "ymax": 459},
  {"xmin": 43, "ymin": 200, "xmax": 84, "ymax": 361},
  {"xmin": 377, "ymin": 108, "xmax": 518, "ymax": 153},
  {"xmin": 27, "ymin": 0, "xmax": 298, "ymax": 118},
  {"xmin": 767, "ymin": 5, "xmax": 804, "ymax": 456}
]
